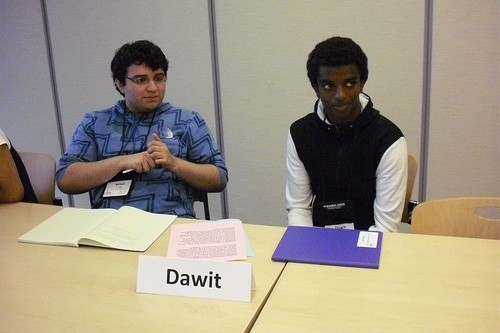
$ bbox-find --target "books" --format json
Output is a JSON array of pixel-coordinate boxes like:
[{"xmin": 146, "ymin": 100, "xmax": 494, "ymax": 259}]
[
  {"xmin": 17, "ymin": 205, "xmax": 178, "ymax": 253},
  {"xmin": 271, "ymin": 226, "xmax": 383, "ymax": 269}
]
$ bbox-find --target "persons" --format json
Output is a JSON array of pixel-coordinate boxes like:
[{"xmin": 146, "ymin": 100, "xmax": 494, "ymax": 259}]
[
  {"xmin": 286, "ymin": 36, "xmax": 408, "ymax": 233},
  {"xmin": 56, "ymin": 40, "xmax": 228, "ymax": 219},
  {"xmin": 0, "ymin": 127, "xmax": 39, "ymax": 204}
]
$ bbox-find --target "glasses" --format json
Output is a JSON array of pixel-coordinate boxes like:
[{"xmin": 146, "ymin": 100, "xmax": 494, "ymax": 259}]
[{"xmin": 124, "ymin": 74, "xmax": 168, "ymax": 85}]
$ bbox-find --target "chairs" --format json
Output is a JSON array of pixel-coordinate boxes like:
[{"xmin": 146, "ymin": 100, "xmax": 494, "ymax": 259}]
[
  {"xmin": 18, "ymin": 152, "xmax": 56, "ymax": 205},
  {"xmin": 401, "ymin": 153, "xmax": 500, "ymax": 240}
]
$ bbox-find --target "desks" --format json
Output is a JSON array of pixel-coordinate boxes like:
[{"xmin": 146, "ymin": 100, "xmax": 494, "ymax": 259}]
[{"xmin": 0, "ymin": 201, "xmax": 500, "ymax": 333}]
